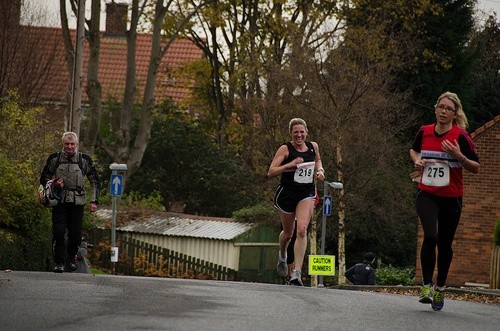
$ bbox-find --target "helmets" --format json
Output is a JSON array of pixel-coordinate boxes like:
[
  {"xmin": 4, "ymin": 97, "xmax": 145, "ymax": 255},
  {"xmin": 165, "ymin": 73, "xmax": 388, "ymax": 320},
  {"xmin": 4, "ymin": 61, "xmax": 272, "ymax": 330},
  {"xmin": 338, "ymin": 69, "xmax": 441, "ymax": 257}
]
[{"xmin": 38, "ymin": 178, "xmax": 64, "ymax": 208}]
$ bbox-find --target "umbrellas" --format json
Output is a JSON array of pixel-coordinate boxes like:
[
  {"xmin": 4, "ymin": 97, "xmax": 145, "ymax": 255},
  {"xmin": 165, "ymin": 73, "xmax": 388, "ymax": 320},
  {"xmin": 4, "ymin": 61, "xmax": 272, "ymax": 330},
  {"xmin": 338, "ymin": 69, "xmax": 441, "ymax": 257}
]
[{"xmin": 343, "ymin": 259, "xmax": 377, "ymax": 285}]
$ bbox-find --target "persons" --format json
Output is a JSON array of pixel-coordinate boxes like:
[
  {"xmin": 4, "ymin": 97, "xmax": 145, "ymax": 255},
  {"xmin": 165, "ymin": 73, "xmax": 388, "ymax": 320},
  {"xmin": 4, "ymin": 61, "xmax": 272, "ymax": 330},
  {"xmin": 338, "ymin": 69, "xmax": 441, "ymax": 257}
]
[
  {"xmin": 410, "ymin": 92, "xmax": 480, "ymax": 311},
  {"xmin": 267, "ymin": 117, "xmax": 326, "ymax": 287},
  {"xmin": 40, "ymin": 132, "xmax": 100, "ymax": 272}
]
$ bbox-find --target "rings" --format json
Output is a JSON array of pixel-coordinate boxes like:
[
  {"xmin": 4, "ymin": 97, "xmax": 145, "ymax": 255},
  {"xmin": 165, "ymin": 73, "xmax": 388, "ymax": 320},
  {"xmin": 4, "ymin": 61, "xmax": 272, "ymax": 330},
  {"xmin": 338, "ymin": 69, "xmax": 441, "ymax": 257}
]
[{"xmin": 446, "ymin": 148, "xmax": 448, "ymax": 150}]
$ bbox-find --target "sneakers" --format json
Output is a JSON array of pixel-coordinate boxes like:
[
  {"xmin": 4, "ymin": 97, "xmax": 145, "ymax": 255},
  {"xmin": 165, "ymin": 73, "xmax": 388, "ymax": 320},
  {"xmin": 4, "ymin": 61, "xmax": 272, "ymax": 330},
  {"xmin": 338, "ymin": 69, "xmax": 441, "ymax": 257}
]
[
  {"xmin": 431, "ymin": 284, "xmax": 445, "ymax": 311},
  {"xmin": 277, "ymin": 250, "xmax": 288, "ymax": 278},
  {"xmin": 288, "ymin": 269, "xmax": 303, "ymax": 286},
  {"xmin": 419, "ymin": 281, "xmax": 433, "ymax": 303}
]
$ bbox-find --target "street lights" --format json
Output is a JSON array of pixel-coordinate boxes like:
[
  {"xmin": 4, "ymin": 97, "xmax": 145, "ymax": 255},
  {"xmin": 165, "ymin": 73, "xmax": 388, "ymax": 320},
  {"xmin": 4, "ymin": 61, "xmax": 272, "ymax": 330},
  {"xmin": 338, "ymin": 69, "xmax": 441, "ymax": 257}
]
[
  {"xmin": 318, "ymin": 180, "xmax": 343, "ymax": 288},
  {"xmin": 108, "ymin": 162, "xmax": 128, "ymax": 275}
]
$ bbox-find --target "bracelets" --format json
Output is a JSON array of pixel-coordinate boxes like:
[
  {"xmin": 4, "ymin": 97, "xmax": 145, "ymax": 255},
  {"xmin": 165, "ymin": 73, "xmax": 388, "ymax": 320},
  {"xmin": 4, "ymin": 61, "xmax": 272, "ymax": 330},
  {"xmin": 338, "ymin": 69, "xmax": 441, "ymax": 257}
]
[{"xmin": 460, "ymin": 156, "xmax": 468, "ymax": 164}]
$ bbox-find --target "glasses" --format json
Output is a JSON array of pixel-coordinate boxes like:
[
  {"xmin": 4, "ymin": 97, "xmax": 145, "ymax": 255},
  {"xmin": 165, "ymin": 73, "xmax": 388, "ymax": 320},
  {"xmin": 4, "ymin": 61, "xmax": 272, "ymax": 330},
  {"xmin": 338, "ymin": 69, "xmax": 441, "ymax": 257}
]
[{"xmin": 436, "ymin": 105, "xmax": 456, "ymax": 112}]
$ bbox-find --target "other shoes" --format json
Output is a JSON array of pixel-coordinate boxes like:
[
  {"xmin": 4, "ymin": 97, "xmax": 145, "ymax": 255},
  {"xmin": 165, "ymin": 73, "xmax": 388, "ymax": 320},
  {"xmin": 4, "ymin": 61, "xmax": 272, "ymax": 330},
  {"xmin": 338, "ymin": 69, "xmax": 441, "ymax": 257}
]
[
  {"xmin": 70, "ymin": 255, "xmax": 78, "ymax": 271},
  {"xmin": 53, "ymin": 263, "xmax": 65, "ymax": 273}
]
